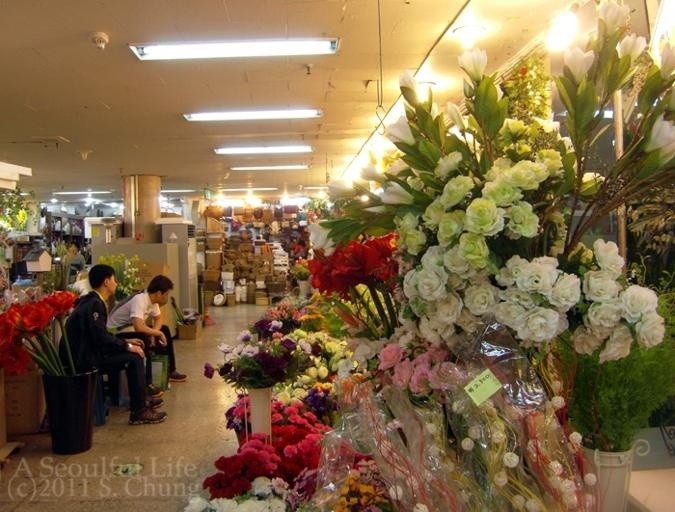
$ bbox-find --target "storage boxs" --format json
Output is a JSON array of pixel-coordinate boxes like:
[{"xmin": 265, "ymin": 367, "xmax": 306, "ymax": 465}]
[{"xmin": 178, "ymin": 323, "xmax": 198, "ymax": 340}]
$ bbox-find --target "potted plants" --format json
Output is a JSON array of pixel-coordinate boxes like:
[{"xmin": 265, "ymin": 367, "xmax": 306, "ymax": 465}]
[{"xmin": 557, "ymin": 253, "xmax": 674, "ymax": 512}]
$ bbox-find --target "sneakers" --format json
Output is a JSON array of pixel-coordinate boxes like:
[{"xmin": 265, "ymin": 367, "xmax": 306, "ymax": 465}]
[
  {"xmin": 128, "ymin": 408, "xmax": 167, "ymax": 425},
  {"xmin": 146, "ymin": 398, "xmax": 164, "ymax": 408},
  {"xmin": 170, "ymin": 372, "xmax": 187, "ymax": 382},
  {"xmin": 147, "ymin": 384, "xmax": 164, "ymax": 398}
]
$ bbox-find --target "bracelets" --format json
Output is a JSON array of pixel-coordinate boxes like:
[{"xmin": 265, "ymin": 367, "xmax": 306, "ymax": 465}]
[{"xmin": 127, "ymin": 343, "xmax": 132, "ymax": 352}]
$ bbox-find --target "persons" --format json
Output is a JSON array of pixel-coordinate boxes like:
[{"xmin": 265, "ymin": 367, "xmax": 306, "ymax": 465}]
[
  {"xmin": 106, "ymin": 274, "xmax": 189, "ymax": 398},
  {"xmin": 59, "ymin": 263, "xmax": 168, "ymax": 426}
]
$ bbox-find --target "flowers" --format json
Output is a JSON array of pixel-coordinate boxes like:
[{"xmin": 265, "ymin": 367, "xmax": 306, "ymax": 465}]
[
  {"xmin": 225, "ymin": 392, "xmax": 336, "ymax": 437},
  {"xmin": 202, "ymin": 432, "xmax": 336, "ymax": 497},
  {"xmin": 50, "ymin": 238, "xmax": 72, "ymax": 293},
  {"xmin": 2, "ymin": 284, "xmax": 43, "ymax": 312},
  {"xmin": 204, "ymin": 317, "xmax": 314, "ymax": 390},
  {"xmin": 98, "ymin": 253, "xmax": 149, "ymax": 303},
  {"xmin": 307, "ymin": 230, "xmax": 402, "ymax": 339},
  {"xmin": 0, "ymin": 257, "xmax": 13, "ymax": 291},
  {"xmin": 181, "ymin": 477, "xmax": 295, "ymax": 512},
  {"xmin": 0, "ymin": 225, "xmax": 18, "ymax": 249},
  {"xmin": 0, "ymin": 187, "xmax": 36, "ymax": 234},
  {"xmin": 308, "ymin": 32, "xmax": 675, "ymax": 362},
  {"xmin": 0, "ymin": 288, "xmax": 79, "ymax": 378}
]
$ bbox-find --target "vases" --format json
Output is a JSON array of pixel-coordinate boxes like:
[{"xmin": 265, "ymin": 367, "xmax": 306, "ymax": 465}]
[
  {"xmin": 42, "ymin": 366, "xmax": 100, "ymax": 456},
  {"xmin": 242, "ymin": 386, "xmax": 272, "ymax": 446}
]
